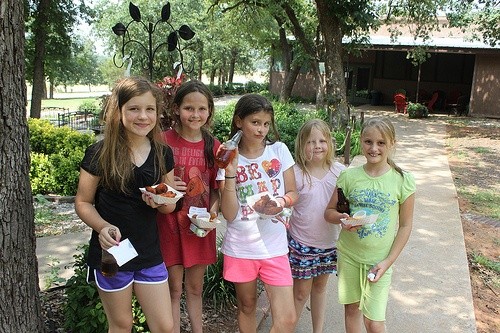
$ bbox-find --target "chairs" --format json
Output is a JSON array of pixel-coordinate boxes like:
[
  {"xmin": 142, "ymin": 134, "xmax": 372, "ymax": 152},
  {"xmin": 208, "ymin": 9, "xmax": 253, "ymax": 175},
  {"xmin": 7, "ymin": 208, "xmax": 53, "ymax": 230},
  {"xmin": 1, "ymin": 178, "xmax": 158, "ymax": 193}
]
[
  {"xmin": 428, "ymin": 93, "xmax": 438, "ymax": 113},
  {"xmin": 394, "ymin": 95, "xmax": 409, "ymax": 114}
]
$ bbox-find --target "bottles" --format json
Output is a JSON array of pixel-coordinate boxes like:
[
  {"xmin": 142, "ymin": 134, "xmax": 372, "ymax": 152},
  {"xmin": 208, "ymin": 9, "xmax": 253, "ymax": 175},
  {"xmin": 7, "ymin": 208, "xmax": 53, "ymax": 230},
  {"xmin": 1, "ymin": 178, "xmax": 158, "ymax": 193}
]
[
  {"xmin": 214, "ymin": 129, "xmax": 244, "ymax": 169},
  {"xmin": 336, "ymin": 187, "xmax": 350, "ymax": 215},
  {"xmin": 101, "ymin": 227, "xmax": 118, "ymax": 279}
]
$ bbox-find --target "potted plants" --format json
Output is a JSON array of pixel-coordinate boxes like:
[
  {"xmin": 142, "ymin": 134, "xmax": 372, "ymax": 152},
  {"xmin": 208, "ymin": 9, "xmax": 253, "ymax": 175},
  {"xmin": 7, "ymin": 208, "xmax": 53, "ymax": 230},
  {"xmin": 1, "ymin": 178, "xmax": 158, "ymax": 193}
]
[{"xmin": 407, "ymin": 101, "xmax": 429, "ymax": 119}]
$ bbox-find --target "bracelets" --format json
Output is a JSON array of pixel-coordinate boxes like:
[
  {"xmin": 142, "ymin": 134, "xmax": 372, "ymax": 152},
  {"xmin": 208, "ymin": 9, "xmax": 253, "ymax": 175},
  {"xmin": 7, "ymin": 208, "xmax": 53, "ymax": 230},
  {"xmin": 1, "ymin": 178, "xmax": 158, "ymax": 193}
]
[
  {"xmin": 275, "ymin": 197, "xmax": 286, "ymax": 208},
  {"xmin": 283, "ymin": 194, "xmax": 292, "ymax": 208},
  {"xmin": 225, "ymin": 175, "xmax": 238, "ymax": 179},
  {"xmin": 223, "ymin": 185, "xmax": 236, "ymax": 192}
]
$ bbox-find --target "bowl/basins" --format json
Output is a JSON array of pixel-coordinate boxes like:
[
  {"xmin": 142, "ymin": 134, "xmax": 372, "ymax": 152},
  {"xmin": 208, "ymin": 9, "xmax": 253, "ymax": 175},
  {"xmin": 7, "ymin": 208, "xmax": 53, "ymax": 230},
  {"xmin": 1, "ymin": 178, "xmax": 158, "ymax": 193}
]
[
  {"xmin": 245, "ymin": 190, "xmax": 284, "ymax": 218},
  {"xmin": 340, "ymin": 214, "xmax": 377, "ymax": 226},
  {"xmin": 139, "ymin": 182, "xmax": 183, "ymax": 204},
  {"xmin": 186, "ymin": 212, "xmax": 221, "ymax": 229},
  {"xmin": 353, "ymin": 211, "xmax": 366, "ymax": 219}
]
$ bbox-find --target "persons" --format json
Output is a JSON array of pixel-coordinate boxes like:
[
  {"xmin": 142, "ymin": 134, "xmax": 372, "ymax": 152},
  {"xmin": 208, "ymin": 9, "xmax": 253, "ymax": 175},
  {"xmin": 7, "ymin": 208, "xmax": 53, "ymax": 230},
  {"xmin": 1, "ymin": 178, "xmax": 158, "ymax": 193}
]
[
  {"xmin": 74, "ymin": 76, "xmax": 177, "ymax": 333},
  {"xmin": 324, "ymin": 115, "xmax": 416, "ymax": 333},
  {"xmin": 153, "ymin": 79, "xmax": 222, "ymax": 333},
  {"xmin": 216, "ymin": 93, "xmax": 299, "ymax": 333},
  {"xmin": 282, "ymin": 118, "xmax": 346, "ymax": 333}
]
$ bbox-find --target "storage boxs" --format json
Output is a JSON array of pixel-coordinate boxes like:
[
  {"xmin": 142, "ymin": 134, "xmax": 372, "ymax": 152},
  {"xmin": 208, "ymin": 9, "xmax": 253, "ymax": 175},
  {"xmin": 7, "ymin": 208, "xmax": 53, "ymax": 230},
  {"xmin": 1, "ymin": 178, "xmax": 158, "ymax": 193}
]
[
  {"xmin": 247, "ymin": 191, "xmax": 284, "ymax": 218},
  {"xmin": 139, "ymin": 183, "xmax": 183, "ymax": 204},
  {"xmin": 340, "ymin": 213, "xmax": 379, "ymax": 227},
  {"xmin": 187, "ymin": 214, "xmax": 221, "ymax": 228}
]
